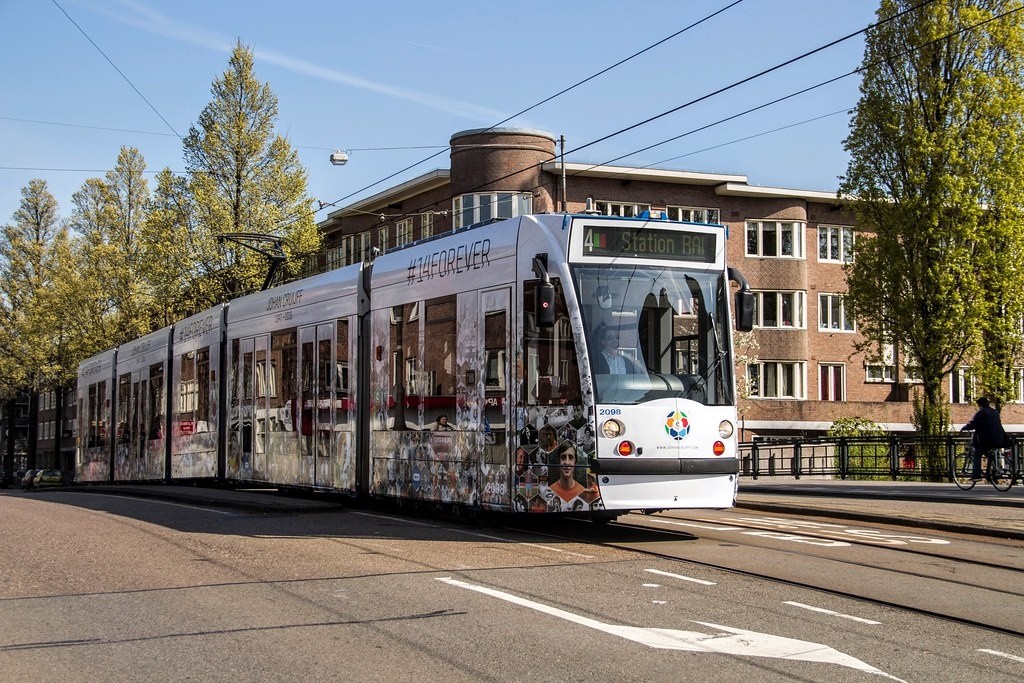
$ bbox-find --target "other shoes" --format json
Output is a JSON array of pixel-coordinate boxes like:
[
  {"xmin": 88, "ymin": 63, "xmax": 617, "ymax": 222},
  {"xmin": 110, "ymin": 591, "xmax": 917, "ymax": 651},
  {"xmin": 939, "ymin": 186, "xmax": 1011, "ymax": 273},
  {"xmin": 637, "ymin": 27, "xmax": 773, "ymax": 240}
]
[{"xmin": 969, "ymin": 477, "xmax": 982, "ymax": 481}]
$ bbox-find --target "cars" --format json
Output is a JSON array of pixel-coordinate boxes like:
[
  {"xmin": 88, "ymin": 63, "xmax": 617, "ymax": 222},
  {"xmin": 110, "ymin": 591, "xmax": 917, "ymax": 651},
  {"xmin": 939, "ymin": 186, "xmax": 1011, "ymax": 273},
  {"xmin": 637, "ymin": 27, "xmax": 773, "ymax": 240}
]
[
  {"xmin": 20, "ymin": 469, "xmax": 40, "ymax": 489},
  {"xmin": 33, "ymin": 469, "xmax": 64, "ymax": 489}
]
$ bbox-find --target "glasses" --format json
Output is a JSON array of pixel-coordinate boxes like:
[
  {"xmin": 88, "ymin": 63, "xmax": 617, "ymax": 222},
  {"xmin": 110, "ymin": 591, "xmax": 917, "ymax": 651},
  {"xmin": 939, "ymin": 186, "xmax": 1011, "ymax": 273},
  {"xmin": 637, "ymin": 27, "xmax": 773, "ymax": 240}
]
[{"xmin": 604, "ymin": 335, "xmax": 619, "ymax": 341}]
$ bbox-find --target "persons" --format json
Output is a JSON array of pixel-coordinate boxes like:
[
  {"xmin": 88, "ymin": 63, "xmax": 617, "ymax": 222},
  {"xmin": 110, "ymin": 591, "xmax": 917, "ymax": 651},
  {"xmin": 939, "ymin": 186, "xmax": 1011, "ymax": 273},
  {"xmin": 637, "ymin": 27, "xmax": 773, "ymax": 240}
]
[
  {"xmin": 373, "ymin": 405, "xmax": 605, "ymax": 511},
  {"xmin": 594, "ymin": 327, "xmax": 637, "ymax": 374},
  {"xmin": 961, "ymin": 397, "xmax": 1006, "ymax": 482}
]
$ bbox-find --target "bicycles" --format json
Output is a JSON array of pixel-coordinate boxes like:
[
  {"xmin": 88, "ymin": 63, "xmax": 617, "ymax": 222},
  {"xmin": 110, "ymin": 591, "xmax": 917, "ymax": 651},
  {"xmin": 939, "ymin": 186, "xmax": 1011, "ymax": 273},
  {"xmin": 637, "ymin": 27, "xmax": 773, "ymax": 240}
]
[{"xmin": 952, "ymin": 429, "xmax": 1015, "ymax": 492}]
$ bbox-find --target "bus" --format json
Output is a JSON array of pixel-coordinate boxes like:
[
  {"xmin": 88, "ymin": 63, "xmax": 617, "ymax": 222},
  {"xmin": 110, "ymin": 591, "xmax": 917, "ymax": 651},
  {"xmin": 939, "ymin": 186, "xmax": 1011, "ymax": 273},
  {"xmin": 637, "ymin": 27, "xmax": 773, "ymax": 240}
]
[{"xmin": 74, "ymin": 210, "xmax": 756, "ymax": 524}]
